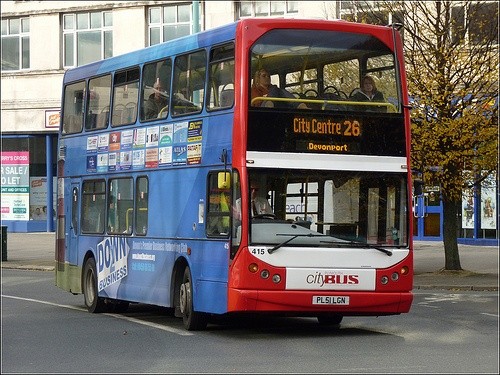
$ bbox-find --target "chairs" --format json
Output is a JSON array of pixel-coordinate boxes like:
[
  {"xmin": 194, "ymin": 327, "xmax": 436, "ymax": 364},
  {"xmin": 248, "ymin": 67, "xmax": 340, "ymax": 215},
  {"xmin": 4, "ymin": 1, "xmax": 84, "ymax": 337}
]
[
  {"xmin": 220, "ymin": 82, "xmax": 234, "ymax": 107},
  {"xmin": 100, "ymin": 99, "xmax": 147, "ymax": 127},
  {"xmin": 292, "ymin": 86, "xmax": 361, "ymax": 111}
]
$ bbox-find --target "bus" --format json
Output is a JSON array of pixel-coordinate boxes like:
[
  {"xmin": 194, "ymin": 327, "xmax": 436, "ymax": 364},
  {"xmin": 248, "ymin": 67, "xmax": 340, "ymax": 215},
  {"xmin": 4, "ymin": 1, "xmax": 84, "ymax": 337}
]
[{"xmin": 55, "ymin": 17, "xmax": 426, "ymax": 332}]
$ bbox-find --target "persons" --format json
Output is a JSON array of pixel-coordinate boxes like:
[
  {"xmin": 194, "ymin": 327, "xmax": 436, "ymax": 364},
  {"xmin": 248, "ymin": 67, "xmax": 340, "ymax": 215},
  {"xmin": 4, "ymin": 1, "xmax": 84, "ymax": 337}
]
[
  {"xmin": 99, "ymin": 195, "xmax": 119, "ymax": 233},
  {"xmin": 178, "ymin": 89, "xmax": 198, "ymax": 113},
  {"xmin": 145, "ymin": 82, "xmax": 184, "ymax": 119},
  {"xmin": 31, "ymin": 206, "xmax": 56, "ymax": 220},
  {"xmin": 233, "ymin": 178, "xmax": 274, "ymax": 223},
  {"xmin": 252, "ymin": 69, "xmax": 311, "ymax": 109},
  {"xmin": 352, "ymin": 76, "xmax": 387, "ymax": 112}
]
